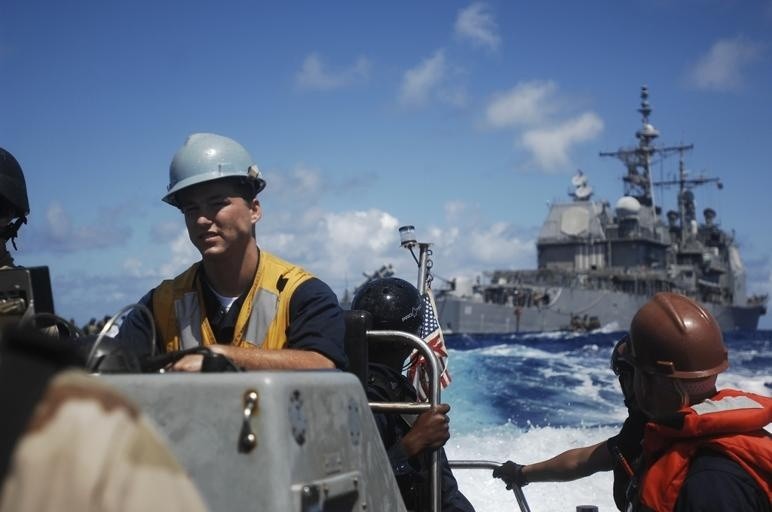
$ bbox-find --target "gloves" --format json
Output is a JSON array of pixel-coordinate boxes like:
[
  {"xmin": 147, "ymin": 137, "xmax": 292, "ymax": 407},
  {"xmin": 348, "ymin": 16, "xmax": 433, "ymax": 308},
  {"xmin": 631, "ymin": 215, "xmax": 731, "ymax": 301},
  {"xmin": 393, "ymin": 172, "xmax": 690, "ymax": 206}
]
[{"xmin": 493, "ymin": 462, "xmax": 528, "ymax": 490}]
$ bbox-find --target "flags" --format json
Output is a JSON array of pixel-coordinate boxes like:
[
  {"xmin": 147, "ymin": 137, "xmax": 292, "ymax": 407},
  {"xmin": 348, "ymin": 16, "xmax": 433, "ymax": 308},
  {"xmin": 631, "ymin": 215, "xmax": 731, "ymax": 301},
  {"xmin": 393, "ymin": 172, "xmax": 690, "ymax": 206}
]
[{"xmin": 413, "ymin": 287, "xmax": 452, "ymax": 402}]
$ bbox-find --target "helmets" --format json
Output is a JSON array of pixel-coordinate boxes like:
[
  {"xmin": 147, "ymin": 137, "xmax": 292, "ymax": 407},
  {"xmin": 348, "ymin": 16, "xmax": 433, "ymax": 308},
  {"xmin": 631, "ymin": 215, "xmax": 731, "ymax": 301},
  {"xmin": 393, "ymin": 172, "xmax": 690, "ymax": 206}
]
[
  {"xmin": 0, "ymin": 149, "xmax": 29, "ymax": 226},
  {"xmin": 351, "ymin": 278, "xmax": 426, "ymax": 338},
  {"xmin": 610, "ymin": 292, "xmax": 728, "ymax": 379},
  {"xmin": 162, "ymin": 133, "xmax": 266, "ymax": 206}
]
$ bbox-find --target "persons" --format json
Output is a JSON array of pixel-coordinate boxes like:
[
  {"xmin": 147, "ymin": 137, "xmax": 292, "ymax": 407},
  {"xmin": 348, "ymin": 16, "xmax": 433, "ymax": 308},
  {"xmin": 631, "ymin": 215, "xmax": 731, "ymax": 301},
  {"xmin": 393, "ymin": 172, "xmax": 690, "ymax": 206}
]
[
  {"xmin": 493, "ymin": 291, "xmax": 772, "ymax": 510},
  {"xmin": 0, "ymin": 149, "xmax": 209, "ymax": 510},
  {"xmin": 345, "ymin": 276, "xmax": 477, "ymax": 511},
  {"xmin": 88, "ymin": 131, "xmax": 354, "ymax": 370}
]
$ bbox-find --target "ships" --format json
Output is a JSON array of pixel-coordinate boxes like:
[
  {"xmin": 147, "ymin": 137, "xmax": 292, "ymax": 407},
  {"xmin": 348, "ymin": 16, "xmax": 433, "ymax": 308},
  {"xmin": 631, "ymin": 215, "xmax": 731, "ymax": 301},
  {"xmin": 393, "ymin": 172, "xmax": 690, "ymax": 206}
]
[{"xmin": 432, "ymin": 86, "xmax": 766, "ymax": 333}]
